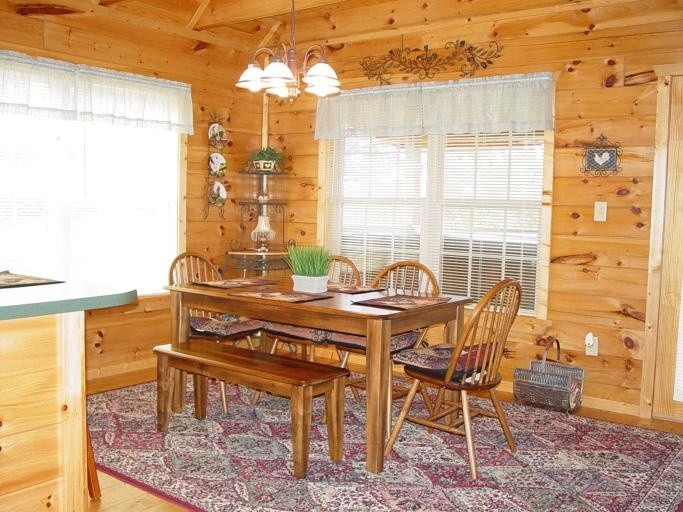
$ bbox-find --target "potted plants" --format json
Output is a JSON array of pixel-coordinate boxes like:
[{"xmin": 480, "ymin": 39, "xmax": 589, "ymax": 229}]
[
  {"xmin": 281, "ymin": 244, "xmax": 335, "ymax": 294},
  {"xmin": 249, "ymin": 146, "xmax": 284, "ymax": 176}
]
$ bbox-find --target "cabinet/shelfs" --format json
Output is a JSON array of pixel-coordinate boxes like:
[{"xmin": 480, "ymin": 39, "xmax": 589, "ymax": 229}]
[{"xmin": 237, "ymin": 172, "xmax": 289, "ymax": 206}]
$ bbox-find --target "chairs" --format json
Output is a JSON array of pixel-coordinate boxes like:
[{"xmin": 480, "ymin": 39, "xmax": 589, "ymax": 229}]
[
  {"xmin": 318, "ymin": 259, "xmax": 442, "ymax": 426},
  {"xmin": 165, "ymin": 250, "xmax": 266, "ymax": 415},
  {"xmin": 252, "ymin": 253, "xmax": 363, "ymax": 411},
  {"xmin": 382, "ymin": 278, "xmax": 523, "ymax": 481}
]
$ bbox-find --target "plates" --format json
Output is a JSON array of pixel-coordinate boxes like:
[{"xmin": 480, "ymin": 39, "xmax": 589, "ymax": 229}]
[
  {"xmin": 206, "ymin": 181, "xmax": 227, "ymax": 207},
  {"xmin": 208, "ymin": 123, "xmax": 227, "ymax": 150},
  {"xmin": 207, "ymin": 152, "xmax": 228, "ymax": 177}
]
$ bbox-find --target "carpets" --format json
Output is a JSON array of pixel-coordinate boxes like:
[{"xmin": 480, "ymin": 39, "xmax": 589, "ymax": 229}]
[{"xmin": 86, "ymin": 369, "xmax": 682, "ymax": 512}]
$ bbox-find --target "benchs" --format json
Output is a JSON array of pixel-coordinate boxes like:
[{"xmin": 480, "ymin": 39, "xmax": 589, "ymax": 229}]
[{"xmin": 151, "ymin": 333, "xmax": 351, "ymax": 479}]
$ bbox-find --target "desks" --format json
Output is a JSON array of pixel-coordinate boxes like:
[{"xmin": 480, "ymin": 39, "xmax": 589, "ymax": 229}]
[{"xmin": 221, "ymin": 250, "xmax": 291, "ymax": 278}]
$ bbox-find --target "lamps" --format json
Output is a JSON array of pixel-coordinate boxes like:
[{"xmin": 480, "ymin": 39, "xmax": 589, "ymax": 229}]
[
  {"xmin": 233, "ymin": 0, "xmax": 343, "ymax": 107},
  {"xmin": 249, "ymin": 214, "xmax": 276, "ymax": 253}
]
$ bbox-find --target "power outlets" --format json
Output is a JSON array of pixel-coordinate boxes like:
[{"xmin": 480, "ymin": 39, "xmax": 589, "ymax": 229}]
[{"xmin": 584, "ymin": 336, "xmax": 600, "ymax": 356}]
